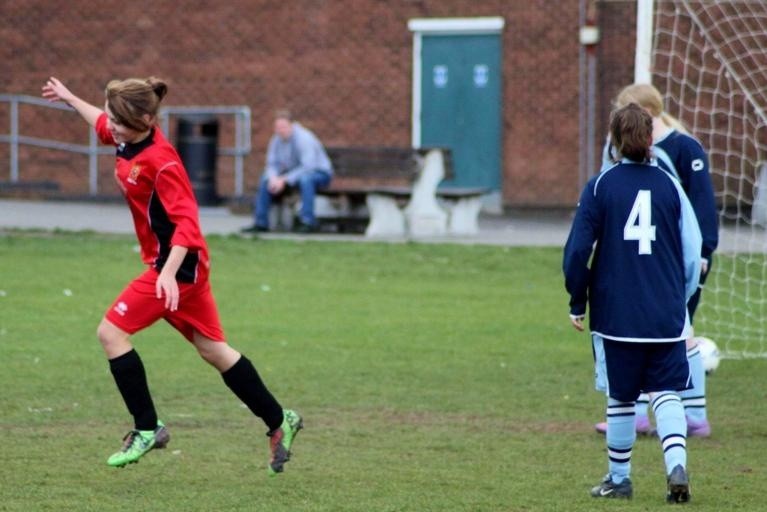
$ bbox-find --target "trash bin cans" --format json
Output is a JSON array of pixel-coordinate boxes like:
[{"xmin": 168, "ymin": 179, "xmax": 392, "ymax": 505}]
[{"xmin": 176, "ymin": 115, "xmax": 220, "ymax": 207}]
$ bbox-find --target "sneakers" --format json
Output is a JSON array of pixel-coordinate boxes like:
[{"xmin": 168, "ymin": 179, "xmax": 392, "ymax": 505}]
[
  {"xmin": 105, "ymin": 420, "xmax": 169, "ymax": 468},
  {"xmin": 589, "ymin": 473, "xmax": 633, "ymax": 499},
  {"xmin": 240, "ymin": 223, "xmax": 321, "ymax": 232},
  {"xmin": 664, "ymin": 464, "xmax": 692, "ymax": 504},
  {"xmin": 594, "ymin": 414, "xmax": 647, "ymax": 435},
  {"xmin": 266, "ymin": 411, "xmax": 304, "ymax": 478},
  {"xmin": 650, "ymin": 418, "xmax": 711, "ymax": 439}
]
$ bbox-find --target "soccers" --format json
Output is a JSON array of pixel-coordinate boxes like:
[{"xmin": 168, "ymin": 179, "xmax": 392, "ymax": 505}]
[{"xmin": 693, "ymin": 335, "xmax": 722, "ymax": 376}]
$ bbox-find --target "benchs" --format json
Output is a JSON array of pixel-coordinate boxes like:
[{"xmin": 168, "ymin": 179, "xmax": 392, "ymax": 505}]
[{"xmin": 247, "ymin": 175, "xmax": 489, "ymax": 233}]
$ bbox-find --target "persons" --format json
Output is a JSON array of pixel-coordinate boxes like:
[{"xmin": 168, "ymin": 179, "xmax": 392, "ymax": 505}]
[
  {"xmin": 560, "ymin": 99, "xmax": 707, "ymax": 505},
  {"xmin": 590, "ymin": 83, "xmax": 721, "ymax": 441},
  {"xmin": 38, "ymin": 76, "xmax": 308, "ymax": 477},
  {"xmin": 238, "ymin": 106, "xmax": 337, "ymax": 236}
]
